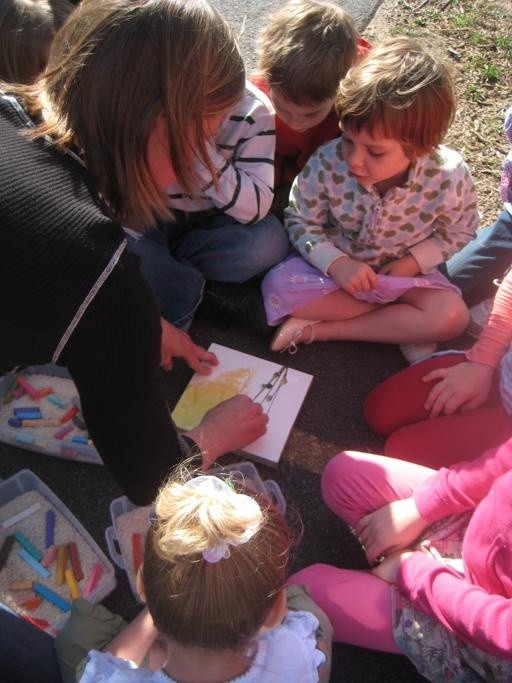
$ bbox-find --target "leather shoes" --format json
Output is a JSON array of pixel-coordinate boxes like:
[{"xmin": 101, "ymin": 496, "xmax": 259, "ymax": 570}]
[{"xmin": 268, "ymin": 317, "xmax": 324, "ymax": 353}]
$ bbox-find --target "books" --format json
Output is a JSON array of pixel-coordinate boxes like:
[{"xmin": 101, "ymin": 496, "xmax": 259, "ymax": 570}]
[{"xmin": 171, "ymin": 343, "xmax": 315, "ymax": 469}]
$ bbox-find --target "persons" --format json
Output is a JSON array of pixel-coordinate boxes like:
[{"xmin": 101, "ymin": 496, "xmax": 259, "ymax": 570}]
[
  {"xmin": 447, "ymin": 102, "xmax": 510, "ymax": 308},
  {"xmin": 261, "ymin": 37, "xmax": 474, "ymax": 360},
  {"xmin": 0, "ymin": 0, "xmax": 268, "ymax": 507},
  {"xmin": 127, "ymin": 79, "xmax": 289, "ymax": 323},
  {"xmin": 246, "ymin": 1, "xmax": 373, "ymax": 227},
  {"xmin": 363, "ymin": 257, "xmax": 509, "ymax": 467},
  {"xmin": 0, "ymin": 1, "xmax": 81, "ymax": 86},
  {"xmin": 71, "ymin": 456, "xmax": 333, "ymax": 683},
  {"xmin": 283, "ymin": 436, "xmax": 509, "ymax": 682}
]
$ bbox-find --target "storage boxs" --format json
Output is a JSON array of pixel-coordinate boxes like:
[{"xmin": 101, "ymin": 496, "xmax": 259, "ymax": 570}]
[
  {"xmin": 1, "ymin": 358, "xmax": 105, "ymax": 464},
  {"xmin": 1, "ymin": 467, "xmax": 118, "ymax": 638},
  {"xmin": 105, "ymin": 461, "xmax": 289, "ymax": 603}
]
